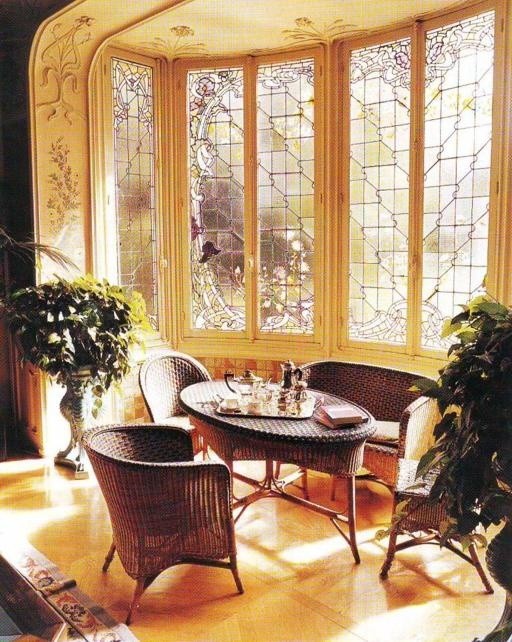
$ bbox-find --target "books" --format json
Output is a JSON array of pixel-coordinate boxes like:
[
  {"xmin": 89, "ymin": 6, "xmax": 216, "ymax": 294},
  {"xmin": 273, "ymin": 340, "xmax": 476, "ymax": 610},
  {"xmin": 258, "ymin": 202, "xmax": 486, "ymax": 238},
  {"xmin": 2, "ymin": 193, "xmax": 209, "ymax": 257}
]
[
  {"xmin": 321, "ymin": 404, "xmax": 362, "ymax": 423},
  {"xmin": 313, "ymin": 406, "xmax": 368, "ymax": 430}
]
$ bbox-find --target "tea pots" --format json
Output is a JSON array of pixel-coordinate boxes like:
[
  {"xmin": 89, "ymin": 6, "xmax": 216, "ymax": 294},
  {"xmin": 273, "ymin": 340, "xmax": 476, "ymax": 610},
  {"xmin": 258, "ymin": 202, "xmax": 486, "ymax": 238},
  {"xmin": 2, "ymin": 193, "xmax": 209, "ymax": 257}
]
[
  {"xmin": 251, "ymin": 379, "xmax": 273, "ymax": 402},
  {"xmin": 279, "ymin": 359, "xmax": 304, "ymax": 398},
  {"xmin": 223, "ymin": 370, "xmax": 274, "ymax": 394}
]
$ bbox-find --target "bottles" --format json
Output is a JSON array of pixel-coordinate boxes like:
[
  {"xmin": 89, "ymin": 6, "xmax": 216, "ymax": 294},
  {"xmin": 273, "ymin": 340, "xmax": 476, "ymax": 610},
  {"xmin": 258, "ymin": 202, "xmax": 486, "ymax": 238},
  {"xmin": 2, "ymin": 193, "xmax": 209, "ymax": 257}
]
[{"xmin": 293, "ymin": 384, "xmax": 306, "ymax": 403}]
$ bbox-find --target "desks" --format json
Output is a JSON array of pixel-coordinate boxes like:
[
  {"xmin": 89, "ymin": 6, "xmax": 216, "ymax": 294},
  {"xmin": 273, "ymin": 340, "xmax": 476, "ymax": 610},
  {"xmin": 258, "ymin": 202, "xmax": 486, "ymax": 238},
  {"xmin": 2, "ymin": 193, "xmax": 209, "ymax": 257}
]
[{"xmin": 177, "ymin": 376, "xmax": 378, "ymax": 566}]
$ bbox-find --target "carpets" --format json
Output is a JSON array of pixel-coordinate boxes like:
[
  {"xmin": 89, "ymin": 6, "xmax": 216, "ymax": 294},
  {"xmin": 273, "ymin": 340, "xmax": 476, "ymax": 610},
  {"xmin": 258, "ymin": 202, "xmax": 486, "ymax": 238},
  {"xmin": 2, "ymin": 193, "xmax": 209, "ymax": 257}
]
[{"xmin": 0, "ymin": 518, "xmax": 145, "ymax": 642}]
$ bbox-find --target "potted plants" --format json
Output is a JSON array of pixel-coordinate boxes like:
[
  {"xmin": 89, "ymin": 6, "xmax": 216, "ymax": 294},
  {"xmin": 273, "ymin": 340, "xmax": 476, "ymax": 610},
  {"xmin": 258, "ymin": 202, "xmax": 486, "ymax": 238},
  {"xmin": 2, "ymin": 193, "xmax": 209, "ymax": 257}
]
[
  {"xmin": 373, "ymin": 290, "xmax": 512, "ymax": 642},
  {"xmin": 0, "ymin": 276, "xmax": 158, "ymax": 470}
]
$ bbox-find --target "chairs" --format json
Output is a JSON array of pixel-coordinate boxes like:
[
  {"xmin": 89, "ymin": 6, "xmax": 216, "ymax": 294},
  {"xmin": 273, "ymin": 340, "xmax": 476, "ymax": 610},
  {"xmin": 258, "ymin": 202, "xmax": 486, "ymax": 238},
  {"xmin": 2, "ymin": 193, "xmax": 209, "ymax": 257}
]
[
  {"xmin": 78, "ymin": 424, "xmax": 244, "ymax": 626},
  {"xmin": 276, "ymin": 359, "xmax": 441, "ymax": 505},
  {"xmin": 379, "ymin": 443, "xmax": 494, "ymax": 596},
  {"xmin": 137, "ymin": 351, "xmax": 215, "ymax": 464}
]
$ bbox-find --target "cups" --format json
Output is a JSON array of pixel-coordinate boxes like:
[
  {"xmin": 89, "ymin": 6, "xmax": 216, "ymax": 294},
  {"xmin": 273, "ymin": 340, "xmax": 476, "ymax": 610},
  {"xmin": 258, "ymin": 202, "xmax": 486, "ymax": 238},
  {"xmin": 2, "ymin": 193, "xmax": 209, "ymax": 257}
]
[{"xmin": 225, "ymin": 397, "xmax": 239, "ymax": 410}]
